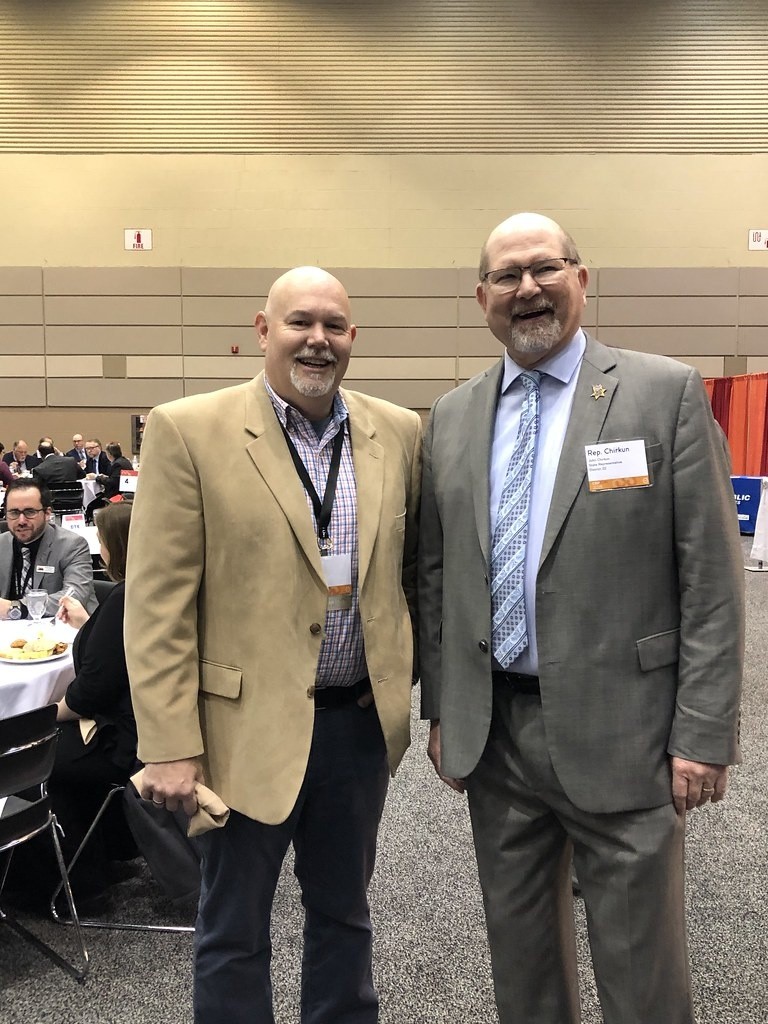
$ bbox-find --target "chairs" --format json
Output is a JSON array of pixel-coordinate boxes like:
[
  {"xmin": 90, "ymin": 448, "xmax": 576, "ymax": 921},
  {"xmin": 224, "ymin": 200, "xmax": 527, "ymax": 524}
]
[
  {"xmin": 14, "ymin": 471, "xmax": 106, "ymax": 517},
  {"xmin": 47, "ymin": 482, "xmax": 85, "ymax": 528},
  {"xmin": 0, "ymin": 703, "xmax": 91, "ymax": 981},
  {"xmin": 51, "ymin": 784, "xmax": 199, "ymax": 934}
]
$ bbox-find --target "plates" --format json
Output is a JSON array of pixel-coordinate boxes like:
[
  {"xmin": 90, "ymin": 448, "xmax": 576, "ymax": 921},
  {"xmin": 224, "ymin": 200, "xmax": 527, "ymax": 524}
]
[{"xmin": 0, "ymin": 646, "xmax": 72, "ymax": 664}]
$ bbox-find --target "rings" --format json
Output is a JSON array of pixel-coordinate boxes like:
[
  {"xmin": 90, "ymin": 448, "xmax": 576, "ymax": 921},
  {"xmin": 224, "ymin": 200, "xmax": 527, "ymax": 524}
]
[
  {"xmin": 702, "ymin": 787, "xmax": 715, "ymax": 792},
  {"xmin": 151, "ymin": 798, "xmax": 165, "ymax": 806}
]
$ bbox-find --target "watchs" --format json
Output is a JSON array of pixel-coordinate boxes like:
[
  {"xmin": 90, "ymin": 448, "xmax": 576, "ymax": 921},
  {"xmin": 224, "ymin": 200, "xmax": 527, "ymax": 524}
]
[{"xmin": 7, "ymin": 600, "xmax": 22, "ymax": 620}]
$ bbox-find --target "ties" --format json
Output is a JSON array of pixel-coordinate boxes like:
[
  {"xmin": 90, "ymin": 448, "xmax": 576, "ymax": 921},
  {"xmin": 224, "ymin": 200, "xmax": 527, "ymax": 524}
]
[
  {"xmin": 490, "ymin": 369, "xmax": 542, "ymax": 671},
  {"xmin": 15, "ymin": 547, "xmax": 32, "ymax": 596}
]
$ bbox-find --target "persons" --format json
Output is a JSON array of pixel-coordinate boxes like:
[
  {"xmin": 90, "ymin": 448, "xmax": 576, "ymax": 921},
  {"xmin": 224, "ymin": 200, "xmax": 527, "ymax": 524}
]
[
  {"xmin": 0, "ymin": 433, "xmax": 134, "ymax": 526},
  {"xmin": 0, "ymin": 476, "xmax": 100, "ymax": 622},
  {"xmin": 0, "ymin": 500, "xmax": 146, "ymax": 917},
  {"xmin": 418, "ymin": 209, "xmax": 745, "ymax": 1024},
  {"xmin": 122, "ymin": 264, "xmax": 424, "ymax": 1024}
]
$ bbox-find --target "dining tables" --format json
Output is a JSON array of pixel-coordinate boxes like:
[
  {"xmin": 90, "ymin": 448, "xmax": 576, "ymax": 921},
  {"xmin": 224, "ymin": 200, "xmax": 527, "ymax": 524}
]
[
  {"xmin": 62, "ymin": 525, "xmax": 103, "ymax": 556},
  {"xmin": 0, "ymin": 616, "xmax": 82, "ymax": 720}
]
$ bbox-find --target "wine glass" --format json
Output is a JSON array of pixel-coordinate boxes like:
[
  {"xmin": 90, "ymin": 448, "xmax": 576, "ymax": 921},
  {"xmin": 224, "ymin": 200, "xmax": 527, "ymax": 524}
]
[{"xmin": 25, "ymin": 587, "xmax": 49, "ymax": 639}]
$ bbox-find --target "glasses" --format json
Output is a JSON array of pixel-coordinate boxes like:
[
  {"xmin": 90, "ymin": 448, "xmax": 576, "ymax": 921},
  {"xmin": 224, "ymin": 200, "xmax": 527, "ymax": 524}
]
[
  {"xmin": 85, "ymin": 446, "xmax": 98, "ymax": 451},
  {"xmin": 479, "ymin": 259, "xmax": 579, "ymax": 297},
  {"xmin": 2, "ymin": 507, "xmax": 47, "ymax": 523}
]
